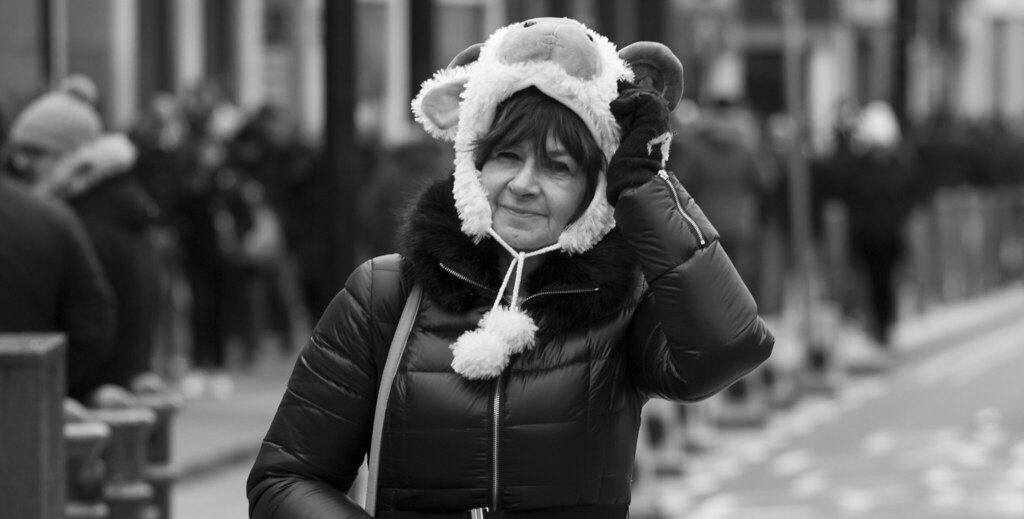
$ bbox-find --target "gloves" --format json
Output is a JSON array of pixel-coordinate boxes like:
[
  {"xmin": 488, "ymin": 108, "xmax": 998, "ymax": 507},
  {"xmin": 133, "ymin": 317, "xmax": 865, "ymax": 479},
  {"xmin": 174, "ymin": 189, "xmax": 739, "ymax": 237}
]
[{"xmin": 604, "ymin": 87, "xmax": 672, "ymax": 209}]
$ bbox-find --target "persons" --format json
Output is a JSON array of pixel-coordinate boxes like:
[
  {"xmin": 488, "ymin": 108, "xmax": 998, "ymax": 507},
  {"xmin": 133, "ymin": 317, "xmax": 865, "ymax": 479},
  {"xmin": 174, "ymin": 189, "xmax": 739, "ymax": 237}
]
[
  {"xmin": 844, "ymin": 99, "xmax": 920, "ymax": 349},
  {"xmin": 0, "ymin": 72, "xmax": 313, "ymax": 422},
  {"xmin": 246, "ymin": 16, "xmax": 796, "ymax": 519}
]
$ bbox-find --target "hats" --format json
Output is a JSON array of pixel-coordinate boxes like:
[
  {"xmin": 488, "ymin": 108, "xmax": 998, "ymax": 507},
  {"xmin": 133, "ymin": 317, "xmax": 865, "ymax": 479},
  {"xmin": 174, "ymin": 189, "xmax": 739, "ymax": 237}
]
[
  {"xmin": 410, "ymin": 16, "xmax": 684, "ymax": 382},
  {"xmin": 7, "ymin": 74, "xmax": 103, "ymax": 162}
]
